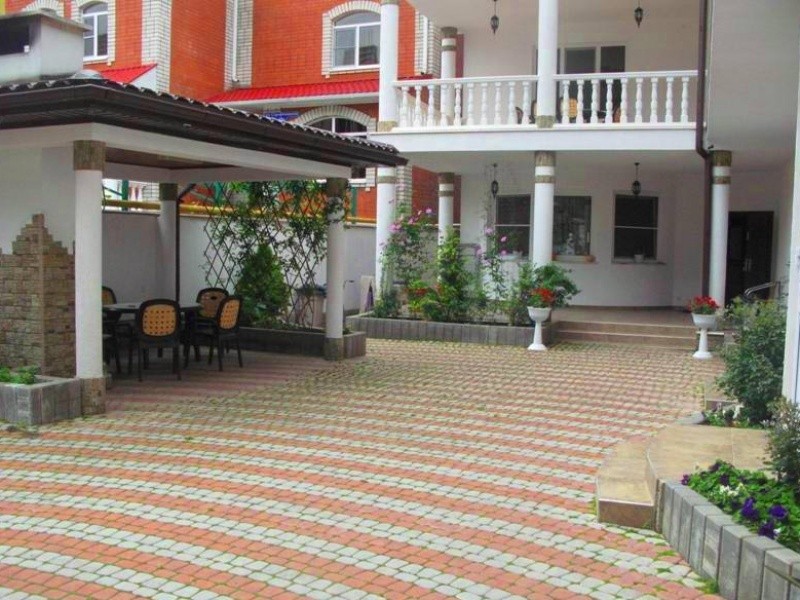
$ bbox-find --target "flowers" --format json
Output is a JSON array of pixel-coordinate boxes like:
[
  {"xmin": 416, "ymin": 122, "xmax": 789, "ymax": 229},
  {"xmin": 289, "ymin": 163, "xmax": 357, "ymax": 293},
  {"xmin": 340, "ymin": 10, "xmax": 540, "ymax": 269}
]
[
  {"xmin": 685, "ymin": 293, "xmax": 722, "ymax": 315},
  {"xmin": 527, "ymin": 285, "xmax": 554, "ymax": 309}
]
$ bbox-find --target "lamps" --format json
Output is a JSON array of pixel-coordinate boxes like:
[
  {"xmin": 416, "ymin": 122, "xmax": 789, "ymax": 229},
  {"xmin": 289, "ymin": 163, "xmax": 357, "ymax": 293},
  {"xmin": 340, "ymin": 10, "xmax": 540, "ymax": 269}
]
[
  {"xmin": 490, "ymin": 0, "xmax": 500, "ymax": 34},
  {"xmin": 491, "ymin": 162, "xmax": 499, "ymax": 199},
  {"xmin": 634, "ymin": 0, "xmax": 644, "ymax": 28},
  {"xmin": 632, "ymin": 162, "xmax": 642, "ymax": 197}
]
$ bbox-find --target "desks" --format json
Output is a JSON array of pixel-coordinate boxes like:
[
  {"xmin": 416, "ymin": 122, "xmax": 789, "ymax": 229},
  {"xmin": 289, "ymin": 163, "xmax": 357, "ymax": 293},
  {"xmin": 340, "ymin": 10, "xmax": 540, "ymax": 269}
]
[
  {"xmin": 102, "ymin": 299, "xmax": 207, "ymax": 371},
  {"xmin": 580, "ymin": 110, "xmax": 609, "ymax": 124}
]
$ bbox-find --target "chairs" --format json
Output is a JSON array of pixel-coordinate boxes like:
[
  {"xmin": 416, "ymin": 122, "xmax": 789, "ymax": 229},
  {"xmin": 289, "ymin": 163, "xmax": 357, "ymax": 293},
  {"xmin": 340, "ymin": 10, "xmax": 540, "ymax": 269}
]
[
  {"xmin": 612, "ymin": 105, "xmax": 620, "ymax": 122},
  {"xmin": 127, "ymin": 298, "xmax": 182, "ymax": 383},
  {"xmin": 558, "ymin": 95, "xmax": 579, "ymax": 124},
  {"xmin": 183, "ymin": 294, "xmax": 246, "ymax": 372},
  {"xmin": 181, "ymin": 287, "xmax": 231, "ymax": 365}
]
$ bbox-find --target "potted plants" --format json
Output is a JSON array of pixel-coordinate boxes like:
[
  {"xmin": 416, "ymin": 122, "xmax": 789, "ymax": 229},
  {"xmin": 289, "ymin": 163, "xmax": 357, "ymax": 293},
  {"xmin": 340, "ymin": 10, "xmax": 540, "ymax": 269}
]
[{"xmin": 633, "ymin": 247, "xmax": 646, "ymax": 263}]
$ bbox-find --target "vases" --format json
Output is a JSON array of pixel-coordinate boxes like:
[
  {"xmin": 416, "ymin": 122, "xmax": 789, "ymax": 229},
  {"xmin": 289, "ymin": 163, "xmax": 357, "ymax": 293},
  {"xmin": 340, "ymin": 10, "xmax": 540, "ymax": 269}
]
[
  {"xmin": 691, "ymin": 311, "xmax": 716, "ymax": 359},
  {"xmin": 527, "ymin": 306, "xmax": 552, "ymax": 350}
]
